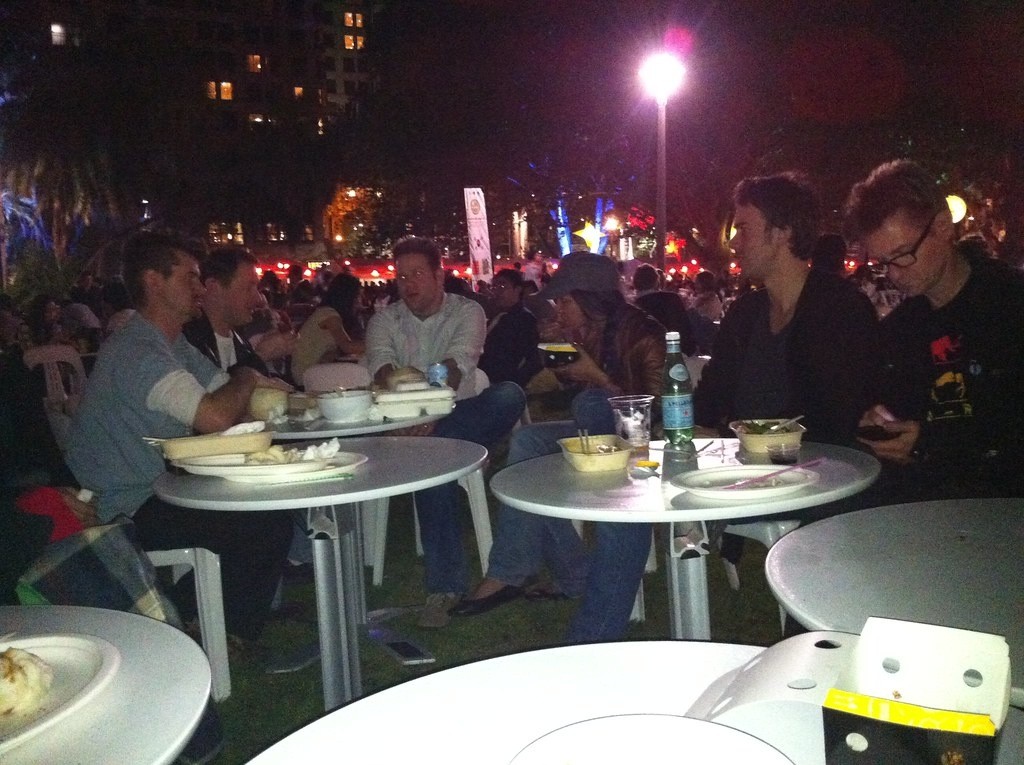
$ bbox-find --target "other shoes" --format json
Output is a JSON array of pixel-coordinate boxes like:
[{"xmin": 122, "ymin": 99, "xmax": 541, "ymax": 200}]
[{"xmin": 448, "ymin": 584, "xmax": 517, "ymax": 616}]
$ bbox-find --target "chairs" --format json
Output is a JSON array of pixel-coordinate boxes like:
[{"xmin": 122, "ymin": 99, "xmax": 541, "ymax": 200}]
[
  {"xmin": 49, "ymin": 412, "xmax": 232, "ymax": 704},
  {"xmin": 570, "ymin": 343, "xmax": 713, "ymax": 618},
  {"xmin": 303, "ymin": 362, "xmax": 493, "ymax": 587},
  {"xmin": 23, "ymin": 346, "xmax": 87, "ymax": 405}
]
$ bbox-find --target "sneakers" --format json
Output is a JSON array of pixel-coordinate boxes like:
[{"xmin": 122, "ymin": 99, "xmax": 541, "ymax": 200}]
[{"xmin": 417, "ymin": 593, "xmax": 460, "ymax": 629}]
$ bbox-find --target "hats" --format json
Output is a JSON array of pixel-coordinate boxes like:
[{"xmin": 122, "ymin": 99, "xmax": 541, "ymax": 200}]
[{"xmin": 528, "ymin": 250, "xmax": 621, "ymax": 300}]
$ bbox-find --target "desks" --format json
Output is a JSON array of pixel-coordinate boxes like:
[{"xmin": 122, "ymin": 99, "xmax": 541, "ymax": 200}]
[{"xmin": 765, "ymin": 497, "xmax": 1024, "ymax": 709}]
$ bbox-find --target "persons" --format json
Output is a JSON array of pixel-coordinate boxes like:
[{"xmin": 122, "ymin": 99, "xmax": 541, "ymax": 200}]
[{"xmin": 0, "ymin": 159, "xmax": 1024, "ymax": 659}]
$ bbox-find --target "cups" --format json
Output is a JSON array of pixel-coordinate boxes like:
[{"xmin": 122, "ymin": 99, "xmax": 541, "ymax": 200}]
[{"xmin": 608, "ymin": 395, "xmax": 656, "ymax": 455}]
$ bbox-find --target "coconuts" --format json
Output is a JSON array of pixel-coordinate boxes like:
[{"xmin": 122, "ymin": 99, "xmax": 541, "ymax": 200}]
[
  {"xmin": 386, "ymin": 366, "xmax": 425, "ymax": 388},
  {"xmin": 248, "ymin": 387, "xmax": 288, "ymax": 419}
]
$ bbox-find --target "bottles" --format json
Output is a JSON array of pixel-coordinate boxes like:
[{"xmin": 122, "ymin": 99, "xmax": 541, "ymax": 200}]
[{"xmin": 660, "ymin": 332, "xmax": 694, "ymax": 442}]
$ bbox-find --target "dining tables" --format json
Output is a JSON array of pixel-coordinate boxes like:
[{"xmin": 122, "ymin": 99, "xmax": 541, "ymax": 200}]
[
  {"xmin": 261, "ymin": 410, "xmax": 452, "ymax": 623},
  {"xmin": 153, "ymin": 436, "xmax": 488, "ymax": 712},
  {"xmin": 489, "ymin": 437, "xmax": 882, "ymax": 641},
  {"xmin": 0, "ymin": 604, "xmax": 211, "ymax": 765},
  {"xmin": 242, "ymin": 641, "xmax": 1023, "ymax": 765}
]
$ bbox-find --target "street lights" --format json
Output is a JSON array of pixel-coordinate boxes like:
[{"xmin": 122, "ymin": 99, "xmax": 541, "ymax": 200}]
[
  {"xmin": 944, "ymin": 194, "xmax": 966, "ymax": 239},
  {"xmin": 639, "ymin": 53, "xmax": 684, "ymax": 270}
]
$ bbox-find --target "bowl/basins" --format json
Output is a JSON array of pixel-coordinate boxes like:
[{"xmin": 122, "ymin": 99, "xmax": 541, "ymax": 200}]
[
  {"xmin": 555, "ymin": 434, "xmax": 635, "ymax": 471},
  {"xmin": 160, "ymin": 431, "xmax": 274, "ymax": 457},
  {"xmin": 730, "ymin": 419, "xmax": 807, "ymax": 464},
  {"xmin": 319, "ymin": 386, "xmax": 454, "ymax": 422},
  {"xmin": 251, "ymin": 386, "xmax": 318, "ymax": 424},
  {"xmin": 537, "ymin": 341, "xmax": 582, "ymax": 367}
]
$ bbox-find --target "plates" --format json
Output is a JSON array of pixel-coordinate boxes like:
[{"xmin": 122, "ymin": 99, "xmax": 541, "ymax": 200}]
[
  {"xmin": 670, "ymin": 465, "xmax": 820, "ymax": 499},
  {"xmin": 172, "ymin": 450, "xmax": 369, "ymax": 483},
  {"xmin": 508, "ymin": 714, "xmax": 795, "ymax": 765},
  {"xmin": 0, "ymin": 633, "xmax": 121, "ymax": 755}
]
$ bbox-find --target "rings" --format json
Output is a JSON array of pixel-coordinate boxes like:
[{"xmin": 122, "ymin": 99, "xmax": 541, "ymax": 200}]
[{"xmin": 562, "ymin": 366, "xmax": 565, "ymax": 373}]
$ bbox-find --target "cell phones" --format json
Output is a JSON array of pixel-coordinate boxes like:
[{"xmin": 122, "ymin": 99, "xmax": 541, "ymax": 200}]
[{"xmin": 854, "ymin": 426, "xmax": 893, "ymax": 442}]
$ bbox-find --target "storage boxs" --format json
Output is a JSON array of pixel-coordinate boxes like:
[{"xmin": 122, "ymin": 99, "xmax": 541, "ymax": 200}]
[
  {"xmin": 537, "ymin": 343, "xmax": 583, "ymax": 368},
  {"xmin": 556, "ymin": 434, "xmax": 634, "ymax": 472},
  {"xmin": 729, "ymin": 419, "xmax": 807, "ymax": 453},
  {"xmin": 689, "ymin": 616, "xmax": 1011, "ymax": 765}
]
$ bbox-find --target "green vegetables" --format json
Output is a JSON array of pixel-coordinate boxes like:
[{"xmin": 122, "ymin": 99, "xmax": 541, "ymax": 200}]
[{"xmin": 743, "ymin": 420, "xmax": 785, "ymax": 434}]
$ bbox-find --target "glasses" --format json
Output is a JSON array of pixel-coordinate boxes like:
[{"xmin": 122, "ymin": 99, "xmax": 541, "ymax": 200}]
[{"xmin": 865, "ymin": 213, "xmax": 938, "ymax": 275}]
